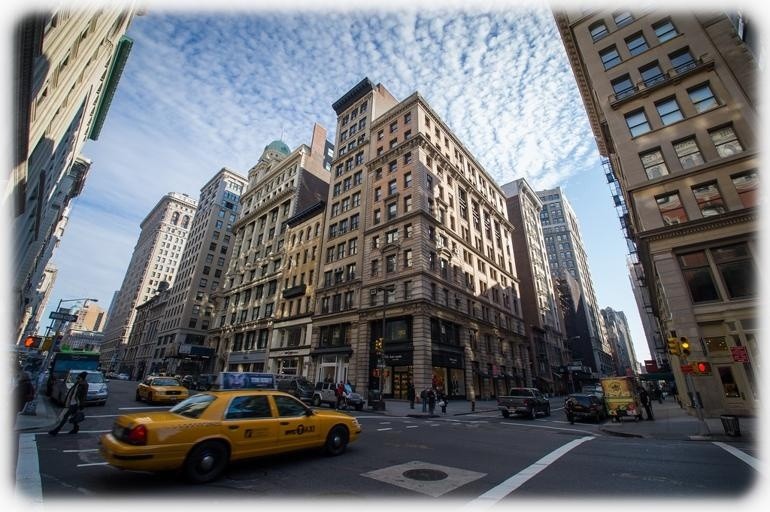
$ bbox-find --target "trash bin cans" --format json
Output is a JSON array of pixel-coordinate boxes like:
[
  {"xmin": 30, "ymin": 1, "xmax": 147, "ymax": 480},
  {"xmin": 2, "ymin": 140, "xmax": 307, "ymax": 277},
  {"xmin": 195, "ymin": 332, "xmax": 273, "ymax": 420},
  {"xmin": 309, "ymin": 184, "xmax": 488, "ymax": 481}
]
[{"xmin": 720, "ymin": 415, "xmax": 741, "ymax": 437}]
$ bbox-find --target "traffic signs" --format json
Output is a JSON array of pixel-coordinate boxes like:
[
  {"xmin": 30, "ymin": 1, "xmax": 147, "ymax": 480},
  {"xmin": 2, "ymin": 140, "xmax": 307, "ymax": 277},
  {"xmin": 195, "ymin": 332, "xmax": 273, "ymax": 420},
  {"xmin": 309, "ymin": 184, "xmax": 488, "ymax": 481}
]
[
  {"xmin": 381, "ymin": 354, "xmax": 403, "ymax": 361},
  {"xmin": 49, "ymin": 312, "xmax": 78, "ymax": 322}
]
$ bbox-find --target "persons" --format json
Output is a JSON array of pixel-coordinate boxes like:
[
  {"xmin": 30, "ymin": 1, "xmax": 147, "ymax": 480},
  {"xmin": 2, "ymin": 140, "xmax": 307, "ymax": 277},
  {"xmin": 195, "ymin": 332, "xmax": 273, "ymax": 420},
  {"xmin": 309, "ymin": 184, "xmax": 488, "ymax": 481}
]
[
  {"xmin": 12, "ymin": 371, "xmax": 34, "ymax": 429},
  {"xmin": 640, "ymin": 387, "xmax": 655, "ymax": 421},
  {"xmin": 46, "ymin": 371, "xmax": 89, "ymax": 436},
  {"xmin": 409, "ymin": 384, "xmax": 449, "ymax": 416},
  {"xmin": 335, "ymin": 383, "xmax": 344, "ymax": 411},
  {"xmin": 567, "ymin": 395, "xmax": 575, "ymax": 425},
  {"xmin": 324, "ymin": 372, "xmax": 333, "ymax": 383}
]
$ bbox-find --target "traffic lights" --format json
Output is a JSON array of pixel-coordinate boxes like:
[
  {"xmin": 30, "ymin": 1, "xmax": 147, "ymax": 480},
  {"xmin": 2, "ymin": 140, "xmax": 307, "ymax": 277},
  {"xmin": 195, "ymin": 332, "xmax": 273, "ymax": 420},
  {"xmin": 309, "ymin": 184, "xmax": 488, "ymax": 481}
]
[
  {"xmin": 667, "ymin": 338, "xmax": 679, "ymax": 356},
  {"xmin": 681, "ymin": 361, "xmax": 714, "ymax": 376},
  {"xmin": 24, "ymin": 337, "xmax": 39, "ymax": 347},
  {"xmin": 375, "ymin": 337, "xmax": 384, "ymax": 352},
  {"xmin": 566, "ymin": 335, "xmax": 580, "ymax": 361},
  {"xmin": 373, "ymin": 368, "xmax": 389, "ymax": 377},
  {"xmin": 681, "ymin": 336, "xmax": 692, "ymax": 355}
]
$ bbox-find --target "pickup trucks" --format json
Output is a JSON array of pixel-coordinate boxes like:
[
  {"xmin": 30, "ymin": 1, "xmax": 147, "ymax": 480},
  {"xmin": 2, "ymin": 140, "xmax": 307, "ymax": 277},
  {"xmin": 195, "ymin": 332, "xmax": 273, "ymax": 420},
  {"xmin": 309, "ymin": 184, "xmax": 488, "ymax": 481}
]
[{"xmin": 498, "ymin": 387, "xmax": 551, "ymax": 420}]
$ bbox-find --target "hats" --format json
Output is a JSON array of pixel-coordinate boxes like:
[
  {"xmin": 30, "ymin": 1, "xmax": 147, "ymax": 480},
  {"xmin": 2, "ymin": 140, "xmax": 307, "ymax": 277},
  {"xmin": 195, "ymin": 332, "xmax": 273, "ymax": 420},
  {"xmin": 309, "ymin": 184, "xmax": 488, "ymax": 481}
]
[{"xmin": 78, "ymin": 370, "xmax": 89, "ymax": 378}]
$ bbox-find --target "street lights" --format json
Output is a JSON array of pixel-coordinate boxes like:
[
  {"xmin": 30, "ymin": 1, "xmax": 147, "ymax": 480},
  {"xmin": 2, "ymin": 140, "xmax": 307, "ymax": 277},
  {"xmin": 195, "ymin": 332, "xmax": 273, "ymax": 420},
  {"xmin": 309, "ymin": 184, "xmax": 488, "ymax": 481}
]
[
  {"xmin": 372, "ymin": 286, "xmax": 396, "ymax": 411},
  {"xmin": 41, "ymin": 298, "xmax": 99, "ymax": 348}
]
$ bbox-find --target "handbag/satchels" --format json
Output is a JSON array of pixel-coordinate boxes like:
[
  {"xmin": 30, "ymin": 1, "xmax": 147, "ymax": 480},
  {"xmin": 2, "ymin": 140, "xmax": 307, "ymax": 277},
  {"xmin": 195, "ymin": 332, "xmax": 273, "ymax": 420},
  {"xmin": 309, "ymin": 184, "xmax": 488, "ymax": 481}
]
[{"xmin": 68, "ymin": 410, "xmax": 85, "ymax": 425}]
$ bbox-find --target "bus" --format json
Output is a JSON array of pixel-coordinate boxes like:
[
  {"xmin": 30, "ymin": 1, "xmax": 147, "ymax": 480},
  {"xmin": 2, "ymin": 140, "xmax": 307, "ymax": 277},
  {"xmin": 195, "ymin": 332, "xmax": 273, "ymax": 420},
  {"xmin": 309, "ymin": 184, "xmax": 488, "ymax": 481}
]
[{"xmin": 46, "ymin": 351, "xmax": 102, "ymax": 395}]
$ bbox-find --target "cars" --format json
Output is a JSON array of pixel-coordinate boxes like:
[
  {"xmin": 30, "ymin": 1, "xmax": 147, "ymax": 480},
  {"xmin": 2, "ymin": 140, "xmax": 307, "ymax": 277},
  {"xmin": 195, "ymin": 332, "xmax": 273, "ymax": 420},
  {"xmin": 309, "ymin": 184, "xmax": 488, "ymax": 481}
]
[{"xmin": 564, "ymin": 377, "xmax": 657, "ymax": 425}]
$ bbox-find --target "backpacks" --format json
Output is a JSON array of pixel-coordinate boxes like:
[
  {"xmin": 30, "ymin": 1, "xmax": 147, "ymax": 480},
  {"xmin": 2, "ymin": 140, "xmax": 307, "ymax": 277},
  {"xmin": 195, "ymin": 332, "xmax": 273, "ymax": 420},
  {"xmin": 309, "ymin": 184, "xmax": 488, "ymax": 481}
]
[{"xmin": 23, "ymin": 381, "xmax": 35, "ymax": 402}]
